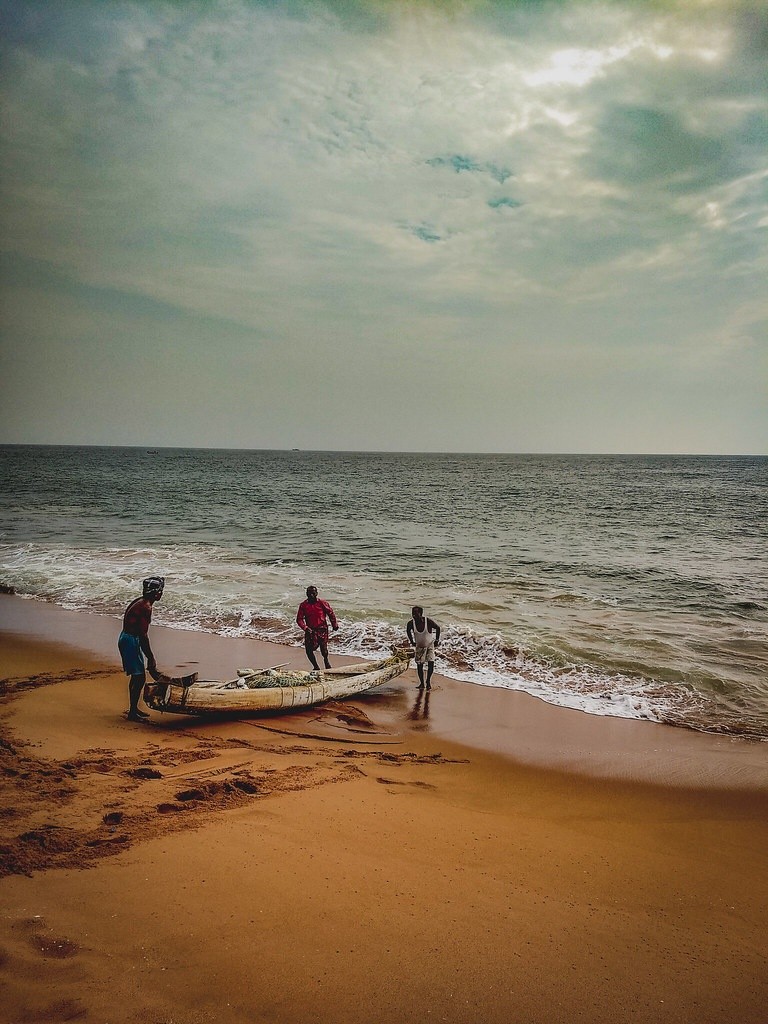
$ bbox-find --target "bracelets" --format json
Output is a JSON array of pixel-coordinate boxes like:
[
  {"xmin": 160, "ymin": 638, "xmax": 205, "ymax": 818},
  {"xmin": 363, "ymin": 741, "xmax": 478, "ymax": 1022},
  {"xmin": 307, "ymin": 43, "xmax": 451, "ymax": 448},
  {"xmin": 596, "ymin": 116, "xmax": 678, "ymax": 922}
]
[{"xmin": 435, "ymin": 639, "xmax": 439, "ymax": 642}]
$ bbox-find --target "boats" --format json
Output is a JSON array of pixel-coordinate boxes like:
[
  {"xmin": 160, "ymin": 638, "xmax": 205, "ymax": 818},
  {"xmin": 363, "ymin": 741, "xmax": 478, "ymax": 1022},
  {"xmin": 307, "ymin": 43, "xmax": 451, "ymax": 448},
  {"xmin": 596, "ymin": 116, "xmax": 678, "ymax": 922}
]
[{"xmin": 141, "ymin": 645, "xmax": 415, "ymax": 717}]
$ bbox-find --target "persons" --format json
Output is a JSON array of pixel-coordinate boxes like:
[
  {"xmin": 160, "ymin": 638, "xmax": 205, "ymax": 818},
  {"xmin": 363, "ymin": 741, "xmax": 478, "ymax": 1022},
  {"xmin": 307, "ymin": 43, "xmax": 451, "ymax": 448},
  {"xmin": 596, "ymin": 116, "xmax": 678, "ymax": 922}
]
[
  {"xmin": 296, "ymin": 585, "xmax": 339, "ymax": 670},
  {"xmin": 407, "ymin": 606, "xmax": 440, "ymax": 689},
  {"xmin": 117, "ymin": 576, "xmax": 164, "ymax": 723}
]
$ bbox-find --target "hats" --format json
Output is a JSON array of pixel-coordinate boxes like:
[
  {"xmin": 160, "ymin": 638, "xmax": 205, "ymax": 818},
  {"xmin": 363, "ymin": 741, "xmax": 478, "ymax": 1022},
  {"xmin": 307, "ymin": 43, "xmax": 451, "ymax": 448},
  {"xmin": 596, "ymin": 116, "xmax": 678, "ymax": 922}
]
[{"xmin": 142, "ymin": 576, "xmax": 165, "ymax": 598}]
